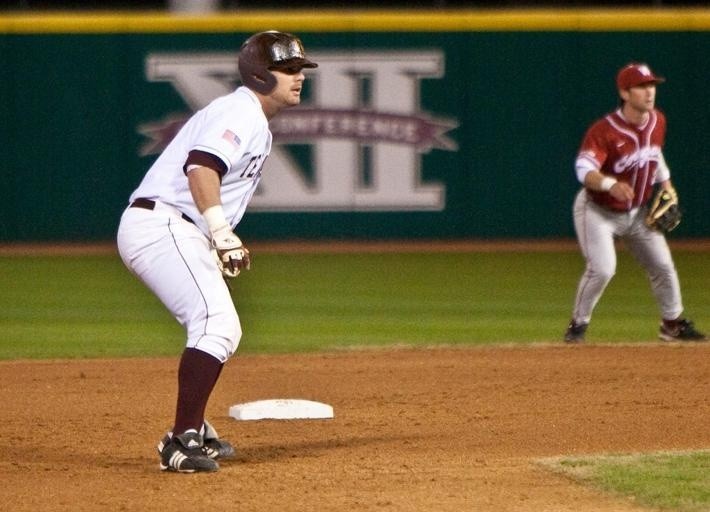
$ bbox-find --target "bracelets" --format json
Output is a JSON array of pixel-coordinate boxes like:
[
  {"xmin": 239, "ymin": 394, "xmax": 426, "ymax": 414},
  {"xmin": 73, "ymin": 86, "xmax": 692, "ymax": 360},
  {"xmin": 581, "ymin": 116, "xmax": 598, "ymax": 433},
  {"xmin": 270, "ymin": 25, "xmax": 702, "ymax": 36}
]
[{"xmin": 601, "ymin": 177, "xmax": 618, "ymax": 193}]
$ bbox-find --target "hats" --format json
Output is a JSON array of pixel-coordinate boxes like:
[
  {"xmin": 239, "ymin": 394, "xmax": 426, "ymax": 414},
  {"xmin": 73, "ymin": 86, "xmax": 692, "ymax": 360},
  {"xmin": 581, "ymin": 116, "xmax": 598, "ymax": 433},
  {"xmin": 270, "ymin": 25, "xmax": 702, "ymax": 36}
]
[{"xmin": 615, "ymin": 63, "xmax": 666, "ymax": 90}]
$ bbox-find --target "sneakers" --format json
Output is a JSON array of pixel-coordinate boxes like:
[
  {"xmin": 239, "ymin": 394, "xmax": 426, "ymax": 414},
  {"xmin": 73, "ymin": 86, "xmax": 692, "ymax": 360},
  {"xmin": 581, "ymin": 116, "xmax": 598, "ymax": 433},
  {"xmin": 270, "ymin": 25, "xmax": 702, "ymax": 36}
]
[
  {"xmin": 158, "ymin": 419, "xmax": 234, "ymax": 473},
  {"xmin": 564, "ymin": 318, "xmax": 589, "ymax": 344},
  {"xmin": 657, "ymin": 318, "xmax": 705, "ymax": 342}
]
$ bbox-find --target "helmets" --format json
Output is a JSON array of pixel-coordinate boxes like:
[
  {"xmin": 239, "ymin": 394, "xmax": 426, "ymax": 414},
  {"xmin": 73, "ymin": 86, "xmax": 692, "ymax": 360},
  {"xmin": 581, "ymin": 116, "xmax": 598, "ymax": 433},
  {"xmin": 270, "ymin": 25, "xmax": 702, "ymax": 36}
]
[{"xmin": 238, "ymin": 30, "xmax": 319, "ymax": 96}]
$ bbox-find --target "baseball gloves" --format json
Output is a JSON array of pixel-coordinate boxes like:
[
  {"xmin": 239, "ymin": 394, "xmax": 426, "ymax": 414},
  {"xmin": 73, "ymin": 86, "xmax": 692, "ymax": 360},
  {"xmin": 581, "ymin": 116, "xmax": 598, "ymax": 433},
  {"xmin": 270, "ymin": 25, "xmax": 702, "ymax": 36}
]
[{"xmin": 645, "ymin": 185, "xmax": 683, "ymax": 234}]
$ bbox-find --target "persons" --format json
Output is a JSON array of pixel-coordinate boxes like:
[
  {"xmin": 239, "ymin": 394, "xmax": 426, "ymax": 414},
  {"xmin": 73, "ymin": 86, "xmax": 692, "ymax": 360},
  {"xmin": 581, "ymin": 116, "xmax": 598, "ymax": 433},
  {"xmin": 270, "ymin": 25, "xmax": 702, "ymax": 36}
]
[
  {"xmin": 563, "ymin": 61, "xmax": 708, "ymax": 345},
  {"xmin": 116, "ymin": 28, "xmax": 319, "ymax": 474}
]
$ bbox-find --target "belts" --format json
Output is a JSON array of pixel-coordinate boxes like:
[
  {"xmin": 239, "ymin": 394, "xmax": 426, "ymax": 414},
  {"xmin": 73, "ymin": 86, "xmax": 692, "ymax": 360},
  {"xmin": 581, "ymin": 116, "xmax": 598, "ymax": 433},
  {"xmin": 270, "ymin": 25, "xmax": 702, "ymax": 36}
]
[{"xmin": 129, "ymin": 198, "xmax": 195, "ymax": 225}]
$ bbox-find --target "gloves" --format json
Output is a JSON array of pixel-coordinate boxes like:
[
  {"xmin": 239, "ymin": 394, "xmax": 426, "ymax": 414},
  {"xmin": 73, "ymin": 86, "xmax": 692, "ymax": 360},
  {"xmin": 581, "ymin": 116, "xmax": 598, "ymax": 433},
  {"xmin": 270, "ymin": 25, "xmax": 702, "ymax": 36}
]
[{"xmin": 209, "ymin": 220, "xmax": 251, "ymax": 278}]
